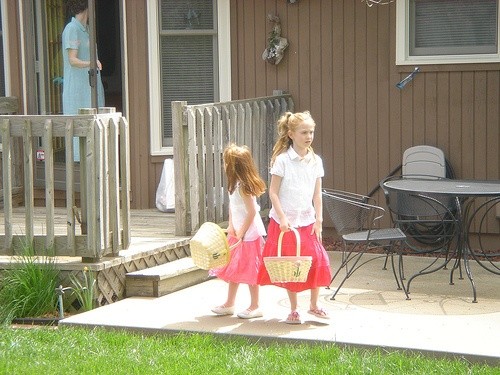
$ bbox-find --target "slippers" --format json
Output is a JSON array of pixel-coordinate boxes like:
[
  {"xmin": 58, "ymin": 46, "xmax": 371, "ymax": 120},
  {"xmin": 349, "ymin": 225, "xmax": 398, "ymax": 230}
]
[
  {"xmin": 286, "ymin": 311, "xmax": 301, "ymax": 323},
  {"xmin": 307, "ymin": 309, "xmax": 330, "ymax": 318}
]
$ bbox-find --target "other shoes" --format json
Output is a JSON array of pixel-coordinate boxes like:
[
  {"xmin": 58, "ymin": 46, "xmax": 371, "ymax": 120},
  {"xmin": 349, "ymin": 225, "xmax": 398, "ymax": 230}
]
[
  {"xmin": 210, "ymin": 304, "xmax": 236, "ymax": 315},
  {"xmin": 237, "ymin": 307, "xmax": 263, "ymax": 319}
]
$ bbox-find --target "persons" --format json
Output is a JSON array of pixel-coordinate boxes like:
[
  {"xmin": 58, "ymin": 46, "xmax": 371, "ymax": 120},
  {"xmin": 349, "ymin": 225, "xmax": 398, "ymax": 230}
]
[
  {"xmin": 257, "ymin": 111, "xmax": 331, "ymax": 324},
  {"xmin": 61, "ymin": 0, "xmax": 105, "ymax": 161},
  {"xmin": 211, "ymin": 145, "xmax": 267, "ymax": 319}
]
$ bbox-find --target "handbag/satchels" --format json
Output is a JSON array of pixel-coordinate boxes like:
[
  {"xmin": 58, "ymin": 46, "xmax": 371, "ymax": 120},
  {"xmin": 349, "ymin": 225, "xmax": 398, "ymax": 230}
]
[{"xmin": 156, "ymin": 158, "xmax": 200, "ymax": 213}]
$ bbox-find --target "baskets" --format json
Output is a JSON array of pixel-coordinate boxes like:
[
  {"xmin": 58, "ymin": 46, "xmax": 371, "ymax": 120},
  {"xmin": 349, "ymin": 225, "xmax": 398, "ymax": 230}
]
[
  {"xmin": 263, "ymin": 227, "xmax": 312, "ymax": 283},
  {"xmin": 190, "ymin": 222, "xmax": 242, "ymax": 270}
]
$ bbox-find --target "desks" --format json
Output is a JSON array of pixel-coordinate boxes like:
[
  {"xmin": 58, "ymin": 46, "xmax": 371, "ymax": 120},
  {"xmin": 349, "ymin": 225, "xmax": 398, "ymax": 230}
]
[{"xmin": 378, "ymin": 178, "xmax": 500, "ymax": 304}]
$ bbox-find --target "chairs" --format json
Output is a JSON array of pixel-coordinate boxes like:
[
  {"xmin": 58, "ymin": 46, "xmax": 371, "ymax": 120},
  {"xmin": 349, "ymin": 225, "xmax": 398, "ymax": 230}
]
[{"xmin": 321, "ymin": 145, "xmax": 478, "ymax": 303}]
